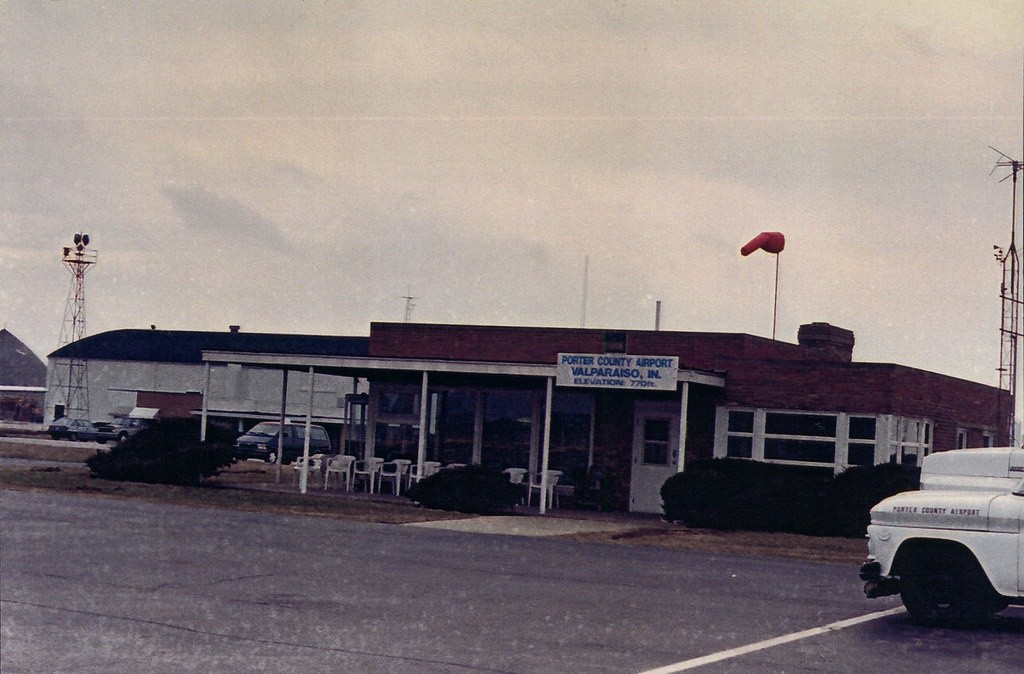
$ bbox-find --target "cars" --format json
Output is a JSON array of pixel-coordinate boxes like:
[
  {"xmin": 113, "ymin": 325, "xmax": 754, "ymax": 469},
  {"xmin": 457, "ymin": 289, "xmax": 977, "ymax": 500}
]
[
  {"xmin": 48, "ymin": 417, "xmax": 100, "ymax": 442},
  {"xmin": 96, "ymin": 417, "xmax": 149, "ymax": 444},
  {"xmin": 858, "ymin": 445, "xmax": 1024, "ymax": 625}
]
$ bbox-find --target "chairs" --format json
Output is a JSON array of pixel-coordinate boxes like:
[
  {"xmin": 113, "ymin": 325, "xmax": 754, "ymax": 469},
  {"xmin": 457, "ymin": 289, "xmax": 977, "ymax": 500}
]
[{"xmin": 292, "ymin": 455, "xmax": 563, "ymax": 509}]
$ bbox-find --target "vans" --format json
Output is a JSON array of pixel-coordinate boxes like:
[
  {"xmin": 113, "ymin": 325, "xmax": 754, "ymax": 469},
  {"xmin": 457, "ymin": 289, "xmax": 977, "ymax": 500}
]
[{"xmin": 233, "ymin": 417, "xmax": 331, "ymax": 466}]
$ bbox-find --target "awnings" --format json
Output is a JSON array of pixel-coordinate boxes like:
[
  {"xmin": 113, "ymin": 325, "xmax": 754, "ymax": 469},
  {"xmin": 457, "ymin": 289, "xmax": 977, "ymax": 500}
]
[{"xmin": 129, "ymin": 407, "xmax": 160, "ymax": 420}]
